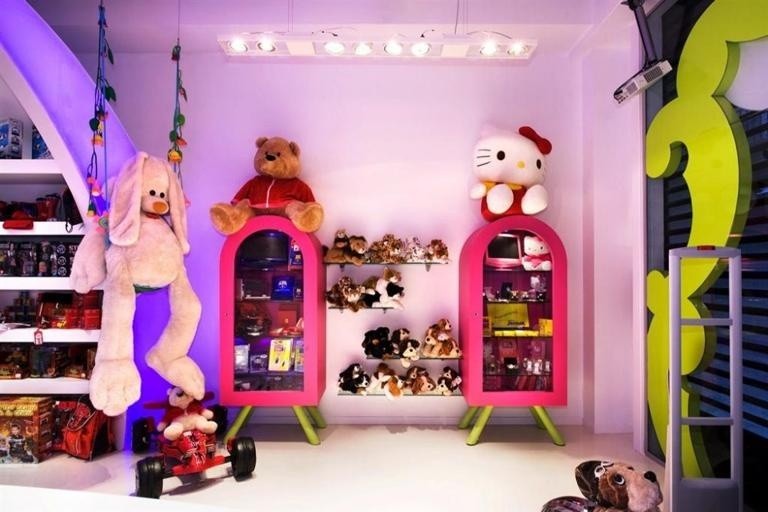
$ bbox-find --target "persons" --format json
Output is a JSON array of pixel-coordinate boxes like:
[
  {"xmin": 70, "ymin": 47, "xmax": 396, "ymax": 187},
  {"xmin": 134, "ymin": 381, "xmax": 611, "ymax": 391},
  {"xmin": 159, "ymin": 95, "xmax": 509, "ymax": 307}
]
[{"xmin": 3, "ymin": 423, "xmax": 37, "ymax": 464}]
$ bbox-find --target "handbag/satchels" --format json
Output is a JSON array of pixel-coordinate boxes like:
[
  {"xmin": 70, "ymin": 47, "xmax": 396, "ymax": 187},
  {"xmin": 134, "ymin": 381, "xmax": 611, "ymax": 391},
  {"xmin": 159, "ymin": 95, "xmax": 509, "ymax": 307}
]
[{"xmin": 51, "ymin": 394, "xmax": 116, "ymax": 462}]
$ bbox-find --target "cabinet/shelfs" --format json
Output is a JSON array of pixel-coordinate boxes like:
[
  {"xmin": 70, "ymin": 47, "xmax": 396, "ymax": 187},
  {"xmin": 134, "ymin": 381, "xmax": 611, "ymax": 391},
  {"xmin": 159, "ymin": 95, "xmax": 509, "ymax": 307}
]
[
  {"xmin": 326, "ymin": 261, "xmax": 447, "ymax": 309},
  {"xmin": 0, "ymin": 158, "xmax": 106, "ymax": 394},
  {"xmin": 459, "ymin": 216, "xmax": 569, "ymax": 446},
  {"xmin": 219, "ymin": 214, "xmax": 327, "ymax": 445},
  {"xmin": 338, "ymin": 357, "xmax": 461, "ymax": 397}
]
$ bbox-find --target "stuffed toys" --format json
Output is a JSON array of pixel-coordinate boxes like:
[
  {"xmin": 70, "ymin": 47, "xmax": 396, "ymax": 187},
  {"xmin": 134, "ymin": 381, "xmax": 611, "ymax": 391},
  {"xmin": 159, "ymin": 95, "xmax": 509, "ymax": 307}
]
[
  {"xmin": 68, "ymin": 148, "xmax": 207, "ymax": 418},
  {"xmin": 468, "ymin": 126, "xmax": 554, "ymax": 221},
  {"xmin": 522, "ymin": 236, "xmax": 552, "ymax": 271},
  {"xmin": 482, "ymin": 275, "xmax": 553, "ymax": 375},
  {"xmin": 154, "ymin": 385, "xmax": 219, "ymax": 438},
  {"xmin": 209, "ymin": 136, "xmax": 324, "ymax": 236},
  {"xmin": 540, "ymin": 460, "xmax": 666, "ymax": 512},
  {"xmin": 322, "ymin": 224, "xmax": 462, "ymax": 397}
]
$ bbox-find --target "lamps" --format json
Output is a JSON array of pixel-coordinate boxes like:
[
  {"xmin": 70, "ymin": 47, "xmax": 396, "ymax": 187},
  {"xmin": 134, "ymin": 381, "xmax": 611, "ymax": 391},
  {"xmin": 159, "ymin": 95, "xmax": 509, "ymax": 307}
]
[{"xmin": 216, "ymin": 0, "xmax": 538, "ymax": 61}]
